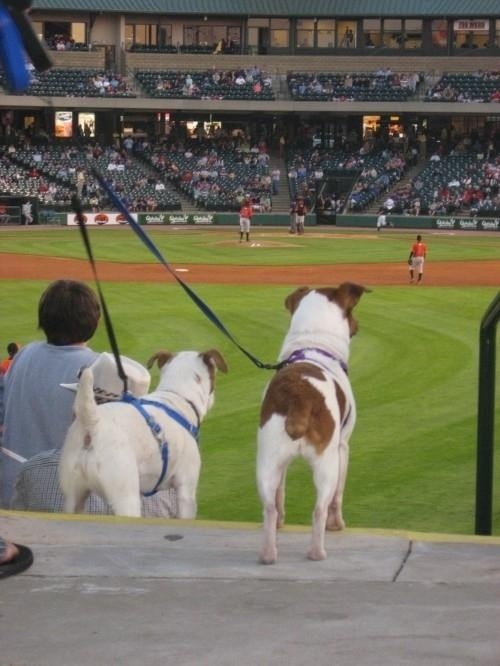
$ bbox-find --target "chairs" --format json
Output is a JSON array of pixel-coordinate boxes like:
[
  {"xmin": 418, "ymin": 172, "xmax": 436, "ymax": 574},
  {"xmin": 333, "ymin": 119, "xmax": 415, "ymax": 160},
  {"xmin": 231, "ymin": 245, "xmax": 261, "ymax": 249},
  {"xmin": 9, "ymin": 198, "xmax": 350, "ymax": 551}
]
[
  {"xmin": 6, "ymin": 67, "xmax": 500, "ymax": 104},
  {"xmin": 126, "ymin": 42, "xmax": 499, "ymax": 56},
  {"xmin": 0, "ymin": 139, "xmax": 499, "ymax": 217}
]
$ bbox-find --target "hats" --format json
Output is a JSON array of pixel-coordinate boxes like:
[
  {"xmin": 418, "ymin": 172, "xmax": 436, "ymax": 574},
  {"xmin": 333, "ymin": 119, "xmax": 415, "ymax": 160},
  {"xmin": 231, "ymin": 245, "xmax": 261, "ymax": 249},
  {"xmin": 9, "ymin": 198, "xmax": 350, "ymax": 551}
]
[{"xmin": 60, "ymin": 353, "xmax": 150, "ymax": 402}]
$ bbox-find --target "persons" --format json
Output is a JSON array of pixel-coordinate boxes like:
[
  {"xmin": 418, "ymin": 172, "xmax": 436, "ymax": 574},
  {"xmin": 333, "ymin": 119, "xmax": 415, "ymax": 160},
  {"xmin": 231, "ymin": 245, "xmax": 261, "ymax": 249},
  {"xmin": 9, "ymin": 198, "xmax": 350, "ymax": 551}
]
[
  {"xmin": 92, "ymin": 69, "xmax": 126, "ymax": 97},
  {"xmin": 0, "ymin": 279, "xmax": 101, "ymax": 505},
  {"xmin": 2, "ymin": 343, "xmax": 21, "ymax": 377},
  {"xmin": 155, "ymin": 63, "xmax": 271, "ymax": 99},
  {"xmin": 0, "ymin": 110, "xmax": 500, "ymax": 225},
  {"xmin": 223, "ymin": 35, "xmax": 234, "ymax": 49},
  {"xmin": 484, "ymin": 39, "xmax": 499, "ymax": 48},
  {"xmin": 408, "ymin": 235, "xmax": 427, "ymax": 284},
  {"xmin": 47, "ymin": 33, "xmax": 76, "ymax": 50},
  {"xmin": 286, "ymin": 65, "xmax": 500, "ymax": 104},
  {"xmin": 461, "ymin": 34, "xmax": 479, "ymax": 48},
  {"xmin": 348, "ymin": 30, "xmax": 353, "ymax": 43},
  {"xmin": 240, "ymin": 199, "xmax": 253, "ymax": 242},
  {"xmin": 376, "ymin": 202, "xmax": 388, "ymax": 232},
  {"xmin": 367, "ymin": 39, "xmax": 375, "ymax": 46},
  {"xmin": 10, "ymin": 353, "xmax": 179, "ymax": 520},
  {"xmin": 289, "ymin": 198, "xmax": 307, "ymax": 236}
]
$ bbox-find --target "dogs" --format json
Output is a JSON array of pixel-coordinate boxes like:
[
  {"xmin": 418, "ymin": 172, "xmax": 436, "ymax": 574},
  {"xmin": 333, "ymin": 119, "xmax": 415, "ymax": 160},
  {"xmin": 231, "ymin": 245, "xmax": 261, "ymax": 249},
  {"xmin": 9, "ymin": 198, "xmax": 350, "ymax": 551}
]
[
  {"xmin": 56, "ymin": 348, "xmax": 228, "ymax": 521},
  {"xmin": 255, "ymin": 280, "xmax": 373, "ymax": 566}
]
